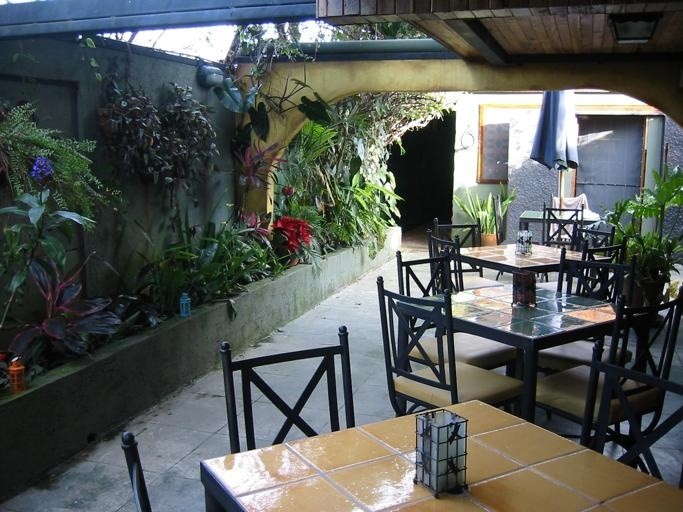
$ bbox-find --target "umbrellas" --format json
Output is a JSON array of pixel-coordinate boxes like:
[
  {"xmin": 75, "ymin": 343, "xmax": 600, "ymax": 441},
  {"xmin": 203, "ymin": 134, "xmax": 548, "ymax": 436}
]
[{"xmin": 531, "ymin": 89, "xmax": 579, "ymax": 250}]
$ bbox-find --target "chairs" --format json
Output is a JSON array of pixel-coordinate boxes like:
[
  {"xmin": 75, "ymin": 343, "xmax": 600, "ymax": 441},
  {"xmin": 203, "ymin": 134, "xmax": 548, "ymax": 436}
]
[
  {"xmin": 426, "ymin": 229, "xmax": 503, "ymax": 336},
  {"xmin": 537, "ymin": 286, "xmax": 682, "ymax": 469},
  {"xmin": 548, "ymin": 195, "xmax": 584, "ymax": 245},
  {"xmin": 375, "ymin": 276, "xmax": 525, "ymax": 418},
  {"xmin": 537, "ymin": 247, "xmax": 636, "ymax": 422},
  {"xmin": 396, "ymin": 246, "xmax": 525, "ymax": 419},
  {"xmin": 434, "ymin": 218, "xmax": 483, "ymax": 276},
  {"xmin": 580, "ymin": 341, "xmax": 683, "ymax": 488},
  {"xmin": 220, "ymin": 326, "xmax": 355, "ymax": 454},
  {"xmin": 566, "ymin": 221, "xmax": 616, "ymax": 288},
  {"xmin": 537, "ymin": 202, "xmax": 577, "ymax": 283},
  {"xmin": 121, "ymin": 431, "xmax": 151, "ymax": 512},
  {"xmin": 536, "ymin": 236, "xmax": 627, "ymax": 302}
]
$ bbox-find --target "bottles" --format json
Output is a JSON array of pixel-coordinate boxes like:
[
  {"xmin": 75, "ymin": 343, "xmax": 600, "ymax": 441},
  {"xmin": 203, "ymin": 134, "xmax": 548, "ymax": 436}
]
[
  {"xmin": 8, "ymin": 356, "xmax": 25, "ymax": 395},
  {"xmin": 179, "ymin": 293, "xmax": 191, "ymax": 318}
]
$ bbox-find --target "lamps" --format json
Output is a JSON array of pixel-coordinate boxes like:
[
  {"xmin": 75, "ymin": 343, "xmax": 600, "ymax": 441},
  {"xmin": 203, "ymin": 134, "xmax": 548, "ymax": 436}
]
[
  {"xmin": 460, "ymin": 125, "xmax": 474, "ymax": 149},
  {"xmin": 607, "ymin": 12, "xmax": 663, "ymax": 44}
]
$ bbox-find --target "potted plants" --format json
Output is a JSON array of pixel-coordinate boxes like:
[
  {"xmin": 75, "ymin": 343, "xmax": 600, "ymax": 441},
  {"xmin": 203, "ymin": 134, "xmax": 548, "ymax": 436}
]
[
  {"xmin": 452, "ymin": 181, "xmax": 520, "ymax": 247},
  {"xmin": 597, "ymin": 163, "xmax": 683, "ymax": 316}
]
